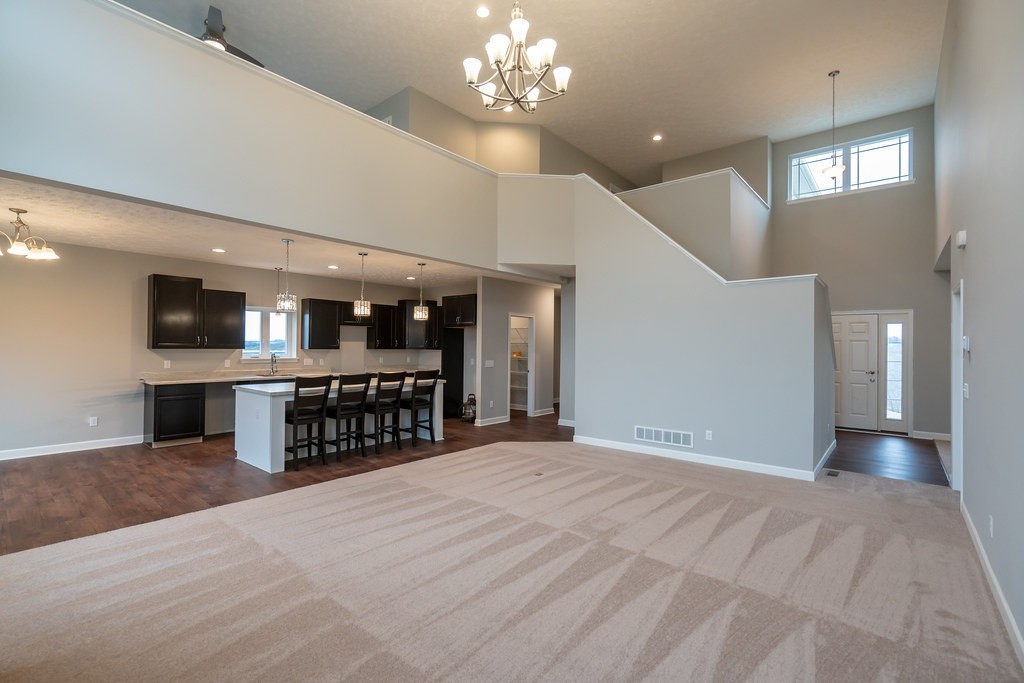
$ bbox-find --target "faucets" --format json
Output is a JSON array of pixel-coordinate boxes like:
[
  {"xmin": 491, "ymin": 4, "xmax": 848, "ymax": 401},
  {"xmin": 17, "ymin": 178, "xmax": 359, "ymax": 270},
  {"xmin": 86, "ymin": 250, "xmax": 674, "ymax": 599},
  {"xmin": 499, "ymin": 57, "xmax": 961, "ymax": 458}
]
[{"xmin": 271, "ymin": 354, "xmax": 278, "ymax": 373}]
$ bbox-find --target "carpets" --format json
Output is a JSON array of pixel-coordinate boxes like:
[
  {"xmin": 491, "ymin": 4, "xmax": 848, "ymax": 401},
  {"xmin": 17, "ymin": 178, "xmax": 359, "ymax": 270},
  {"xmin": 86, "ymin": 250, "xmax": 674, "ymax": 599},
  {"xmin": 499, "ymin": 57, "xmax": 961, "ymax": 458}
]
[{"xmin": 0, "ymin": 442, "xmax": 1024, "ymax": 683}]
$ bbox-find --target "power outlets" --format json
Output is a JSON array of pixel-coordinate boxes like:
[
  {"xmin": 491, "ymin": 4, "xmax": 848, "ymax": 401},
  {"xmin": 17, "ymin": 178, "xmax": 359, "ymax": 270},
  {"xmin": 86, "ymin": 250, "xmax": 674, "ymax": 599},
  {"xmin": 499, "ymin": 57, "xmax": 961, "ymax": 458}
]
[
  {"xmin": 379, "ymin": 357, "xmax": 384, "ymax": 364},
  {"xmin": 164, "ymin": 360, "xmax": 170, "ymax": 369},
  {"xmin": 89, "ymin": 417, "xmax": 98, "ymax": 426},
  {"xmin": 706, "ymin": 431, "xmax": 712, "ymax": 441},
  {"xmin": 319, "ymin": 359, "xmax": 325, "ymax": 366},
  {"xmin": 989, "ymin": 514, "xmax": 995, "ymax": 538},
  {"xmin": 225, "ymin": 360, "xmax": 230, "ymax": 367},
  {"xmin": 304, "ymin": 359, "xmax": 314, "ymax": 366},
  {"xmin": 406, "ymin": 357, "xmax": 411, "ymax": 363}
]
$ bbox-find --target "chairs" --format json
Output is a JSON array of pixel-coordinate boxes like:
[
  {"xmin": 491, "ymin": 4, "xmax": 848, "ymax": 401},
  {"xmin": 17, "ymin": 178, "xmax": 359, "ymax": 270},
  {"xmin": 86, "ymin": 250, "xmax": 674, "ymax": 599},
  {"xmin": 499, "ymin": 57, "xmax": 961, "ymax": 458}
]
[
  {"xmin": 401, "ymin": 370, "xmax": 440, "ymax": 447},
  {"xmin": 367, "ymin": 371, "xmax": 406, "ymax": 453},
  {"xmin": 285, "ymin": 374, "xmax": 333, "ymax": 472},
  {"xmin": 327, "ymin": 373, "xmax": 372, "ymax": 463}
]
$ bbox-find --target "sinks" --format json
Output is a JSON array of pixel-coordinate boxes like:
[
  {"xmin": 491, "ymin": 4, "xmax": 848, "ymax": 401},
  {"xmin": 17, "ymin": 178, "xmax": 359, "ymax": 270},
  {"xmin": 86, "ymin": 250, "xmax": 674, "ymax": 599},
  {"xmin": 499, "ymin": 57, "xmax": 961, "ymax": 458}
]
[{"xmin": 256, "ymin": 374, "xmax": 297, "ymax": 377}]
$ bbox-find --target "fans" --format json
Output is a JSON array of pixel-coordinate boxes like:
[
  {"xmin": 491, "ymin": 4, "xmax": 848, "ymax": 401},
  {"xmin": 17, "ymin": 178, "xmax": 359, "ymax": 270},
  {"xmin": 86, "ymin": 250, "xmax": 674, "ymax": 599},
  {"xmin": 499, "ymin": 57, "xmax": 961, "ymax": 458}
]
[{"xmin": 196, "ymin": 6, "xmax": 265, "ymax": 70}]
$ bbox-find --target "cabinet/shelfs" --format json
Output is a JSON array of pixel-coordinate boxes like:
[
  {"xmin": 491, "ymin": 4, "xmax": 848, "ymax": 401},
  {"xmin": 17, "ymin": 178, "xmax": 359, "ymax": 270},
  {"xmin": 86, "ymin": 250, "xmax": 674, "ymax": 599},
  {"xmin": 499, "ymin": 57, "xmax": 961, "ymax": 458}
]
[
  {"xmin": 301, "ymin": 293, "xmax": 477, "ymax": 352},
  {"xmin": 138, "ymin": 372, "xmax": 206, "ymax": 450},
  {"xmin": 511, "ymin": 327, "xmax": 528, "ymax": 390},
  {"xmin": 145, "ymin": 272, "xmax": 247, "ymax": 351}
]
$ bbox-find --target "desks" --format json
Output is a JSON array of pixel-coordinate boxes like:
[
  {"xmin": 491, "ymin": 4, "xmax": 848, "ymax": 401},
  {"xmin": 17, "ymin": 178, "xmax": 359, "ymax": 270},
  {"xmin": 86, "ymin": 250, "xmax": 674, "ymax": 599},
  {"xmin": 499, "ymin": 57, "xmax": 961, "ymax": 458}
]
[{"xmin": 232, "ymin": 379, "xmax": 447, "ymax": 474}]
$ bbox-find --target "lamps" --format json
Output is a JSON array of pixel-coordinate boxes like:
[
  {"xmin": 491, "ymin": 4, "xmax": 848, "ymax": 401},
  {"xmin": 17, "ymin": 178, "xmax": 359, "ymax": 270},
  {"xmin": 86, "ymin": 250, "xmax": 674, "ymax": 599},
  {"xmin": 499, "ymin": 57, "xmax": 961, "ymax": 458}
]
[
  {"xmin": 413, "ymin": 263, "xmax": 429, "ymax": 321},
  {"xmin": 820, "ymin": 68, "xmax": 847, "ymax": 183},
  {"xmin": 490, "ymin": 401, "xmax": 493, "ymax": 408},
  {"xmin": 463, "ymin": 0, "xmax": 573, "ymax": 114},
  {"xmin": 0, "ymin": 208, "xmax": 61, "ymax": 261},
  {"xmin": 353, "ymin": 252, "xmax": 371, "ymax": 317},
  {"xmin": 199, "ymin": 33, "xmax": 227, "ymax": 52},
  {"xmin": 276, "ymin": 239, "xmax": 298, "ymax": 313},
  {"xmin": 458, "ymin": 394, "xmax": 476, "ymax": 423}
]
